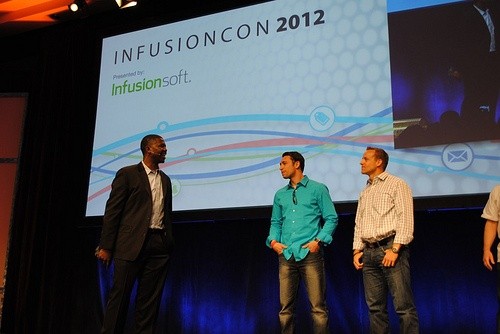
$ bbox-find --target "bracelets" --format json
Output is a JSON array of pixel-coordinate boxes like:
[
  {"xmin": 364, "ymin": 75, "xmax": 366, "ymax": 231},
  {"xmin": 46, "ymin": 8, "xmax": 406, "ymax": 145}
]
[
  {"xmin": 271, "ymin": 241, "xmax": 276, "ymax": 247},
  {"xmin": 353, "ymin": 251, "xmax": 360, "ymax": 255}
]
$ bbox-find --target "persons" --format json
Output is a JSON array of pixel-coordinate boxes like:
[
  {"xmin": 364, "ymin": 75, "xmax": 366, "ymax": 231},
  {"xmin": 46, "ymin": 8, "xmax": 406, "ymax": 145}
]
[
  {"xmin": 395, "ymin": 94, "xmax": 500, "ymax": 148},
  {"xmin": 265, "ymin": 151, "xmax": 339, "ymax": 334},
  {"xmin": 98, "ymin": 134, "xmax": 172, "ymax": 334},
  {"xmin": 481, "ymin": 183, "xmax": 500, "ymax": 334},
  {"xmin": 353, "ymin": 145, "xmax": 418, "ymax": 334}
]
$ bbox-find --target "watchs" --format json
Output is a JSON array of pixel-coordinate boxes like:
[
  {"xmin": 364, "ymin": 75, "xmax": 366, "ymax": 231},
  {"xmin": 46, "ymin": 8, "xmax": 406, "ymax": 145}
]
[
  {"xmin": 391, "ymin": 247, "xmax": 398, "ymax": 253},
  {"xmin": 315, "ymin": 238, "xmax": 322, "ymax": 247}
]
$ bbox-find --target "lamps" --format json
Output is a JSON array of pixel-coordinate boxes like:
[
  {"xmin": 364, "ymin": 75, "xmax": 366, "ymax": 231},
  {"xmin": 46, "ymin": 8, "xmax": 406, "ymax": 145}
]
[{"xmin": 68, "ymin": 0, "xmax": 87, "ymax": 12}]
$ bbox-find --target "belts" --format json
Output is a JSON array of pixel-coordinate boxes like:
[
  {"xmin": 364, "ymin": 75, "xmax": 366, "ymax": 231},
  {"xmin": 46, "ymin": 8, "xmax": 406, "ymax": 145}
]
[{"xmin": 366, "ymin": 234, "xmax": 395, "ymax": 249}]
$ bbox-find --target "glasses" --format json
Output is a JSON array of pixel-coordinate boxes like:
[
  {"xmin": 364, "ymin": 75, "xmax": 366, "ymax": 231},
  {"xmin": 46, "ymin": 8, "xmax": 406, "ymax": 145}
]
[{"xmin": 292, "ymin": 189, "xmax": 297, "ymax": 205}]
